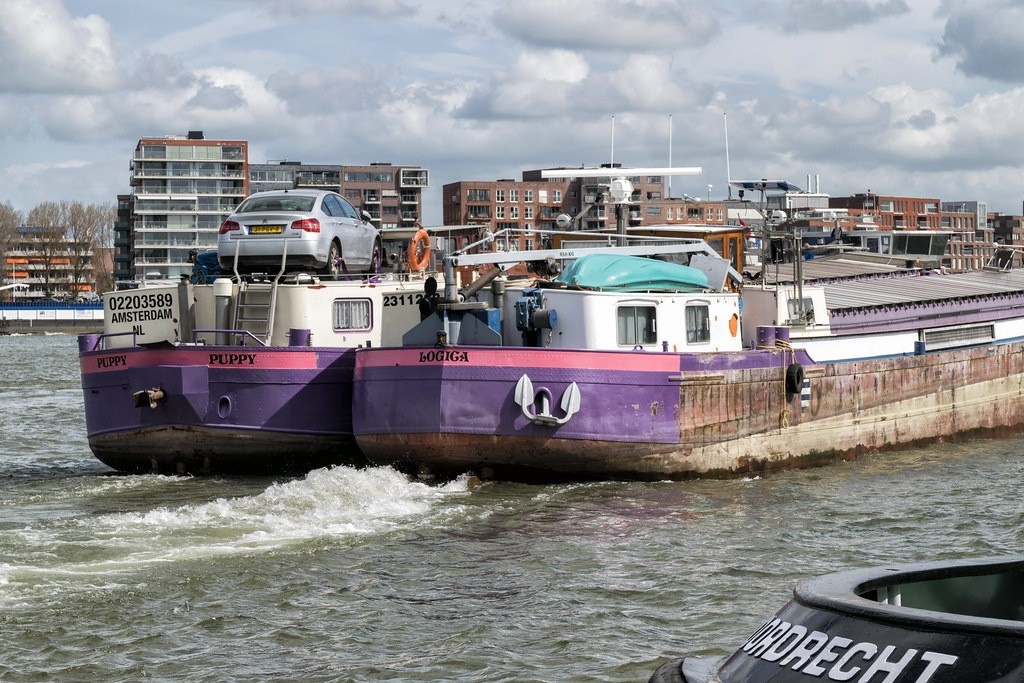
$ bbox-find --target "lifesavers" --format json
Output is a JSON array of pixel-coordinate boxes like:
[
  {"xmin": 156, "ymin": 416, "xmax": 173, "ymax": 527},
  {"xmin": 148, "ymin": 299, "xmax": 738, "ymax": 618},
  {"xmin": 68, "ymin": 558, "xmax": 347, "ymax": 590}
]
[
  {"xmin": 788, "ymin": 363, "xmax": 804, "ymax": 394},
  {"xmin": 408, "ymin": 229, "xmax": 432, "ymax": 270}
]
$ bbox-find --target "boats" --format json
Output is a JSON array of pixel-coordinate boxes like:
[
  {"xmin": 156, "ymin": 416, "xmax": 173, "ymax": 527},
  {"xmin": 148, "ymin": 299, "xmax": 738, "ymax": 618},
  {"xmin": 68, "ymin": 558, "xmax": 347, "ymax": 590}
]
[
  {"xmin": 646, "ymin": 555, "xmax": 1022, "ymax": 683},
  {"xmin": 75, "ymin": 229, "xmax": 1023, "ymax": 480}
]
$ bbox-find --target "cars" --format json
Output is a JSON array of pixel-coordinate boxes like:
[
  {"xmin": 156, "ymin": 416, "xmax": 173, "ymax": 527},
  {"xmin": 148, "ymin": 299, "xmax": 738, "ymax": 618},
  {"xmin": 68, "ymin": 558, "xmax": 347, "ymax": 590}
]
[{"xmin": 214, "ymin": 189, "xmax": 384, "ymax": 276}]
